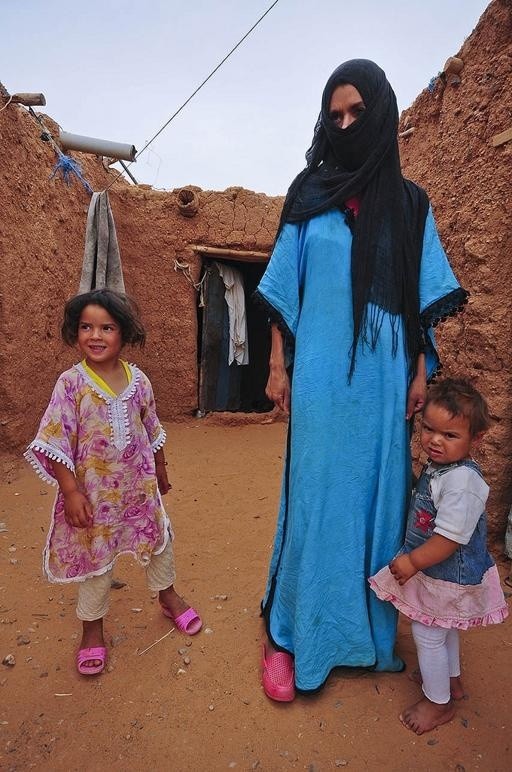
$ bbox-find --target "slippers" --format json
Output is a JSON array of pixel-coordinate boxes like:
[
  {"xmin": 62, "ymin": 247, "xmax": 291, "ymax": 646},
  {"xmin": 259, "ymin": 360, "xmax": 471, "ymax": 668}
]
[
  {"xmin": 77, "ymin": 646, "xmax": 107, "ymax": 675},
  {"xmin": 161, "ymin": 605, "xmax": 201, "ymax": 635}
]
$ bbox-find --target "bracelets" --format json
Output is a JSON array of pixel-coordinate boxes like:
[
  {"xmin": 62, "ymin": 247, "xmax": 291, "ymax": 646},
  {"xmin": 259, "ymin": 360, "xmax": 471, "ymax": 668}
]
[{"xmin": 155, "ymin": 459, "xmax": 169, "ymax": 467}]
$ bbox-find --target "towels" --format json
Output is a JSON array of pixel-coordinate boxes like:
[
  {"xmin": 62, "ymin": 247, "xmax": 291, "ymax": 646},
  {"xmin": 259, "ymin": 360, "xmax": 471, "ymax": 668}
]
[{"xmin": 77, "ymin": 190, "xmax": 125, "ymax": 295}]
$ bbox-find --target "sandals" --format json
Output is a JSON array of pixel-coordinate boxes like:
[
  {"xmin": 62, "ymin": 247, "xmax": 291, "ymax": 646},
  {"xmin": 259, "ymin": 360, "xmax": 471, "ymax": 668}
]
[{"xmin": 261, "ymin": 640, "xmax": 296, "ymax": 702}]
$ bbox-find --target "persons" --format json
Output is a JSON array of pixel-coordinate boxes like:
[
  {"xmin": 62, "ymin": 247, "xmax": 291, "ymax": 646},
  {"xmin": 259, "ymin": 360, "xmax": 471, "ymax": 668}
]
[
  {"xmin": 24, "ymin": 288, "xmax": 203, "ymax": 676},
  {"xmin": 364, "ymin": 377, "xmax": 509, "ymax": 738},
  {"xmin": 254, "ymin": 58, "xmax": 468, "ymax": 703}
]
